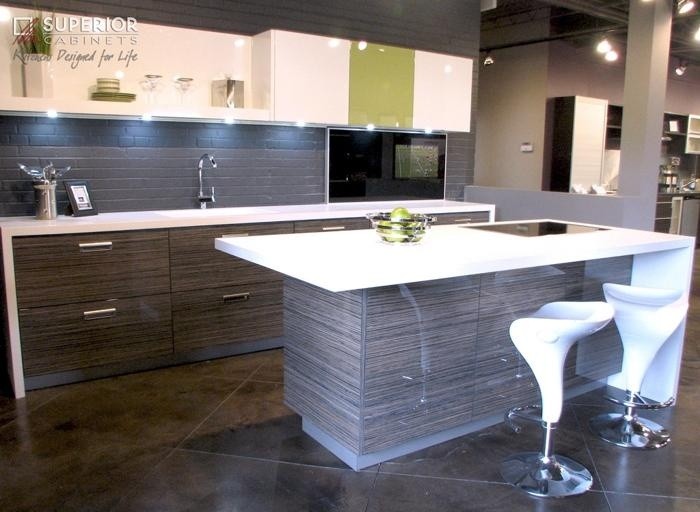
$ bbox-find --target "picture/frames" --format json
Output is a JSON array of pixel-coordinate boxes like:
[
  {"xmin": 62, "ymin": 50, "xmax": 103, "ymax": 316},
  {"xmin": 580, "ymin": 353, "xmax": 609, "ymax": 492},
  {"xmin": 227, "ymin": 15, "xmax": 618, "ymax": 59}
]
[{"xmin": 63, "ymin": 179, "xmax": 98, "ymax": 216}]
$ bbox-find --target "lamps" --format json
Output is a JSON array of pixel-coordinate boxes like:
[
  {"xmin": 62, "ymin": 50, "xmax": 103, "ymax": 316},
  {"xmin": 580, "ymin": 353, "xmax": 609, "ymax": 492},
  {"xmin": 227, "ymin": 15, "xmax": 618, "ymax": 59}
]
[
  {"xmin": 483, "ymin": 55, "xmax": 494, "ymax": 66},
  {"xmin": 597, "ymin": 30, "xmax": 618, "ymax": 61},
  {"xmin": 677, "ymin": 0, "xmax": 696, "ymax": 14},
  {"xmin": 675, "ymin": 60, "xmax": 689, "ymax": 76}
]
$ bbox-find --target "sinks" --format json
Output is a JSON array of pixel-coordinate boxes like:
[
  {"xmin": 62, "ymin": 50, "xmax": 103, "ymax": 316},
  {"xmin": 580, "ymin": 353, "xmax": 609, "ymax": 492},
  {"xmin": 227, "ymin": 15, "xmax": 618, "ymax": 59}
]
[{"xmin": 159, "ymin": 207, "xmax": 256, "ymax": 216}]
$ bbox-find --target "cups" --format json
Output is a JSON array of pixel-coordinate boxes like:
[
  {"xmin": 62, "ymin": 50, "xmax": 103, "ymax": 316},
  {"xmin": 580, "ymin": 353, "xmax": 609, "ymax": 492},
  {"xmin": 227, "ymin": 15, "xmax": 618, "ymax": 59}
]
[
  {"xmin": 33, "ymin": 184, "xmax": 57, "ymax": 219},
  {"xmin": 96, "ymin": 78, "xmax": 120, "ymax": 93}
]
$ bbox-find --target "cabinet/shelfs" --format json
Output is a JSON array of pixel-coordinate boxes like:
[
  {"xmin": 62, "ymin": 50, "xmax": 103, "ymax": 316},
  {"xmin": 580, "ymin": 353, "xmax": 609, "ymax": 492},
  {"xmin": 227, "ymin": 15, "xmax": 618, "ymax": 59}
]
[
  {"xmin": 250, "ymin": 29, "xmax": 474, "ymax": 134},
  {"xmin": 282, "ymin": 256, "xmax": 632, "ymax": 475},
  {"xmin": 549, "ymin": 95, "xmax": 700, "ymax": 246},
  {"xmin": 2, "ymin": 205, "xmax": 495, "ymax": 392}
]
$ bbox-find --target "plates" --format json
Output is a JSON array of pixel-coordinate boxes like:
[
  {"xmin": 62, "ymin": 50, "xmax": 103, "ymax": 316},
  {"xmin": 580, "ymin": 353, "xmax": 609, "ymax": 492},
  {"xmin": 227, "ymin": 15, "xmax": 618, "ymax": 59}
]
[{"xmin": 91, "ymin": 92, "xmax": 137, "ymax": 103}]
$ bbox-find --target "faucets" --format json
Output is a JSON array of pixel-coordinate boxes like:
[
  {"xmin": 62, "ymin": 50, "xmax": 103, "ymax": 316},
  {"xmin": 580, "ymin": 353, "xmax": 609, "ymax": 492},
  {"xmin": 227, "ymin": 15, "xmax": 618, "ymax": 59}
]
[{"xmin": 197, "ymin": 154, "xmax": 217, "ymax": 195}]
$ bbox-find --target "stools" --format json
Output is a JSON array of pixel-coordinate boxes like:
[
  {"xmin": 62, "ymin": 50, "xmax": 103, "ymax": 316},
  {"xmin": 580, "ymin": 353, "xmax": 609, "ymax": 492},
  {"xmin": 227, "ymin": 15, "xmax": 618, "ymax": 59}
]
[
  {"xmin": 588, "ymin": 280, "xmax": 688, "ymax": 451},
  {"xmin": 499, "ymin": 301, "xmax": 614, "ymax": 498}
]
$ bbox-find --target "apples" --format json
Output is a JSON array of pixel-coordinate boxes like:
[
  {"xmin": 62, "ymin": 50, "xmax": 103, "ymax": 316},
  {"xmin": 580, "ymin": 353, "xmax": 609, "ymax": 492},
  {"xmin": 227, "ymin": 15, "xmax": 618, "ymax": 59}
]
[{"xmin": 378, "ymin": 207, "xmax": 426, "ymax": 243}]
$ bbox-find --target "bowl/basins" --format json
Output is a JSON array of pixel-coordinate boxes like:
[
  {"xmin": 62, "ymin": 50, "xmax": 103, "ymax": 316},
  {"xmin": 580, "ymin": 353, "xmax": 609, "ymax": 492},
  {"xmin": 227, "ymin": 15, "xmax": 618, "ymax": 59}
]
[{"xmin": 364, "ymin": 212, "xmax": 437, "ymax": 242}]
